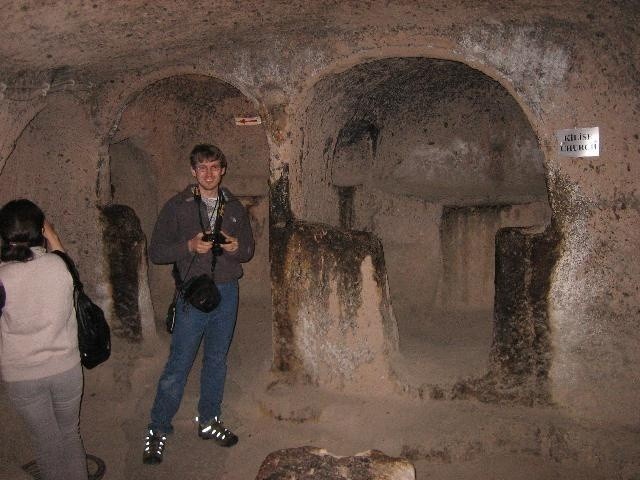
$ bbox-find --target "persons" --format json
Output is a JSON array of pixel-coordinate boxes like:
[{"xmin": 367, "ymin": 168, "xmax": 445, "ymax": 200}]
[
  {"xmin": 142, "ymin": 143, "xmax": 255, "ymax": 463},
  {"xmin": 0, "ymin": 197, "xmax": 89, "ymax": 480}
]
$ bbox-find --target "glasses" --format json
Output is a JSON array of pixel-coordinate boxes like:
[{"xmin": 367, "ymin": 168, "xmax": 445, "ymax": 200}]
[{"xmin": 195, "ymin": 165, "xmax": 220, "ymax": 173}]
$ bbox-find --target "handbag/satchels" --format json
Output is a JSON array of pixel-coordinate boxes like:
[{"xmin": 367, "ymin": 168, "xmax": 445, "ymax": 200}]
[
  {"xmin": 51, "ymin": 248, "xmax": 111, "ymax": 370},
  {"xmin": 184, "ymin": 271, "xmax": 222, "ymax": 312}
]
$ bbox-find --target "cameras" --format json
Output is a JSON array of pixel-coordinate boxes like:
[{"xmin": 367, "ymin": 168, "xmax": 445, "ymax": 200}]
[{"xmin": 202, "ymin": 234, "xmax": 225, "ymax": 256}]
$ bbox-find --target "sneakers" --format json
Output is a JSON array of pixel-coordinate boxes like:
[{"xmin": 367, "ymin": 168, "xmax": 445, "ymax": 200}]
[
  {"xmin": 144, "ymin": 424, "xmax": 167, "ymax": 465},
  {"xmin": 198, "ymin": 418, "xmax": 238, "ymax": 445}
]
